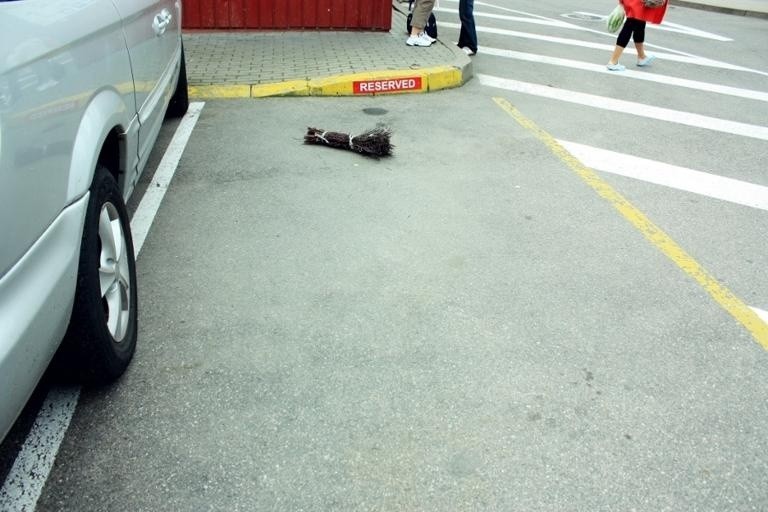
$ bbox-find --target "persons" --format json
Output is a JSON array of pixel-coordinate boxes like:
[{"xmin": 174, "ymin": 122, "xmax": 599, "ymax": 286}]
[
  {"xmin": 455, "ymin": 0, "xmax": 478, "ymax": 55},
  {"xmin": 406, "ymin": 0, "xmax": 437, "ymax": 48},
  {"xmin": 605, "ymin": 0, "xmax": 669, "ymax": 71}
]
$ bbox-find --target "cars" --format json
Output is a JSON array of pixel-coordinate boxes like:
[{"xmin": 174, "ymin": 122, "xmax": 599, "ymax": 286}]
[{"xmin": 0, "ymin": 0, "xmax": 190, "ymax": 448}]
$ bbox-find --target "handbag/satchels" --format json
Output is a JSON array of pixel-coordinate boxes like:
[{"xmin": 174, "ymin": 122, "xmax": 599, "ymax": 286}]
[{"xmin": 406, "ymin": 11, "xmax": 437, "ymax": 38}]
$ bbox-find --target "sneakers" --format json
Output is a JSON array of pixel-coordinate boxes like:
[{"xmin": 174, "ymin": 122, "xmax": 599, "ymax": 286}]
[
  {"xmin": 462, "ymin": 47, "xmax": 474, "ymax": 54},
  {"xmin": 607, "ymin": 60, "xmax": 626, "ymax": 71},
  {"xmin": 420, "ymin": 31, "xmax": 437, "ymax": 43},
  {"xmin": 636, "ymin": 53, "xmax": 654, "ymax": 66},
  {"xmin": 407, "ymin": 35, "xmax": 432, "ymax": 47},
  {"xmin": 619, "ymin": 0, "xmax": 669, "ymax": 25}
]
